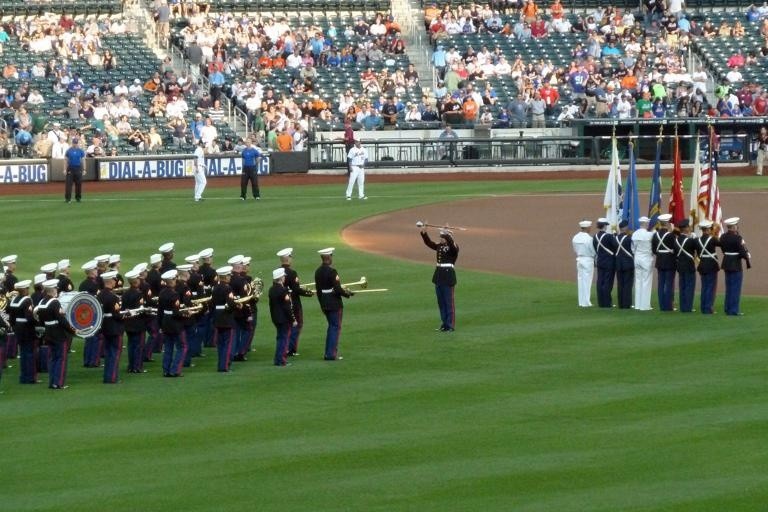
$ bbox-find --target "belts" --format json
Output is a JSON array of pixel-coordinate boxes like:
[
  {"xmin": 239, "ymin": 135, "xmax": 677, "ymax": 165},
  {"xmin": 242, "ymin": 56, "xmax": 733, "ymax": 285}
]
[
  {"xmin": 163, "ymin": 310, "xmax": 173, "ymax": 315},
  {"xmin": 44, "ymin": 320, "xmax": 59, "ymax": 325},
  {"xmin": 14, "ymin": 318, "xmax": 28, "ymax": 322},
  {"xmin": 103, "ymin": 312, "xmax": 115, "ymax": 318},
  {"xmin": 435, "ymin": 262, "xmax": 454, "ymax": 268},
  {"xmin": 724, "ymin": 252, "xmax": 740, "ymax": 256},
  {"xmin": 658, "ymin": 249, "xmax": 673, "ymax": 253},
  {"xmin": 701, "ymin": 253, "xmax": 716, "ymax": 257},
  {"xmin": 320, "ymin": 289, "xmax": 336, "ymax": 293},
  {"xmin": 215, "ymin": 305, "xmax": 227, "ymax": 310}
]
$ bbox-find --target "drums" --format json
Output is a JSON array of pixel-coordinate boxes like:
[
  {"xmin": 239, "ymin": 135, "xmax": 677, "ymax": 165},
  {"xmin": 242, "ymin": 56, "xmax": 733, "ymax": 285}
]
[{"xmin": 57, "ymin": 290, "xmax": 103, "ymax": 338}]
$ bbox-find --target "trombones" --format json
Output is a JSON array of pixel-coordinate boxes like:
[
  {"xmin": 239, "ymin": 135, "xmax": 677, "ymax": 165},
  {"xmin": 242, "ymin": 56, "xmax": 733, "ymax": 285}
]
[{"xmin": 341, "ymin": 276, "xmax": 388, "ymax": 293}]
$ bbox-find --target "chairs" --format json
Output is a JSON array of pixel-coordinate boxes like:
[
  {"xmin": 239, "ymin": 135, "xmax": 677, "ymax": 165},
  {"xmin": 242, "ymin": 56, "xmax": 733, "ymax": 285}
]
[{"xmin": 0, "ymin": 0, "xmax": 767, "ymax": 157}]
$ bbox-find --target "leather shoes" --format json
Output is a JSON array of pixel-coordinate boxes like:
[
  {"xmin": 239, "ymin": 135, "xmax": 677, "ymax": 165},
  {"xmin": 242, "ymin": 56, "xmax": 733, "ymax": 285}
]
[
  {"xmin": 436, "ymin": 323, "xmax": 456, "ymax": 334},
  {"xmin": 577, "ymin": 303, "xmax": 747, "ymax": 317}
]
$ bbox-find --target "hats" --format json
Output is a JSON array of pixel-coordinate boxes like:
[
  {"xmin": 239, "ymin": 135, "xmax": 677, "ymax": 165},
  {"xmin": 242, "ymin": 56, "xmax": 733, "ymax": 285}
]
[
  {"xmin": 0, "ymin": 242, "xmax": 200, "ymax": 290},
  {"xmin": 216, "ymin": 266, "xmax": 233, "ymax": 276},
  {"xmin": 678, "ymin": 219, "xmax": 689, "ymax": 228},
  {"xmin": 199, "ymin": 138, "xmax": 209, "ymax": 144},
  {"xmin": 699, "ymin": 220, "xmax": 713, "ymax": 229},
  {"xmin": 577, "ymin": 220, "xmax": 592, "ymax": 229},
  {"xmin": 316, "ymin": 247, "xmax": 335, "ymax": 256},
  {"xmin": 72, "ymin": 138, "xmax": 79, "ymax": 144},
  {"xmin": 637, "ymin": 217, "xmax": 651, "ymax": 223},
  {"xmin": 199, "ymin": 248, "xmax": 214, "ymax": 259},
  {"xmin": 243, "ymin": 257, "xmax": 251, "ymax": 265},
  {"xmin": 598, "ymin": 217, "xmax": 611, "ymax": 224},
  {"xmin": 227, "ymin": 255, "xmax": 244, "ymax": 264},
  {"xmin": 275, "ymin": 248, "xmax": 293, "ymax": 257},
  {"xmin": 724, "ymin": 216, "xmax": 740, "ymax": 226},
  {"xmin": 657, "ymin": 214, "xmax": 672, "ymax": 222},
  {"xmin": 439, "ymin": 228, "xmax": 453, "ymax": 236},
  {"xmin": 272, "ymin": 268, "xmax": 287, "ymax": 279}
]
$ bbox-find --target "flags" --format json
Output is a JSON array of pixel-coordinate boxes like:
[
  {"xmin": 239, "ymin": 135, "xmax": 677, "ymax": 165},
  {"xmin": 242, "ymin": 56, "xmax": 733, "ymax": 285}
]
[
  {"xmin": 688, "ymin": 134, "xmax": 707, "ymax": 229},
  {"xmin": 698, "ymin": 124, "xmax": 723, "ymax": 223},
  {"xmin": 645, "ymin": 139, "xmax": 662, "ymax": 232},
  {"xmin": 602, "ymin": 134, "xmax": 625, "ymax": 230},
  {"xmin": 667, "ymin": 130, "xmax": 686, "ymax": 225},
  {"xmin": 620, "ymin": 141, "xmax": 640, "ymax": 236}
]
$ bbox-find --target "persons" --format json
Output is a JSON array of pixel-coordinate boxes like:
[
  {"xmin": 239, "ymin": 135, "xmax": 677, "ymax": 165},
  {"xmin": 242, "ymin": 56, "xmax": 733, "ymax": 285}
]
[
  {"xmin": 193, "ymin": 137, "xmax": 209, "ymax": 202},
  {"xmin": 572, "ymin": 220, "xmax": 597, "ymax": 308},
  {"xmin": 63, "ymin": 138, "xmax": 86, "ymax": 203},
  {"xmin": 238, "ymin": 136, "xmax": 264, "ymax": 200},
  {"xmin": 671, "ymin": 218, "xmax": 698, "ymax": 313},
  {"xmin": 719, "ymin": 217, "xmax": 751, "ymax": 316},
  {"xmin": 416, "ymin": 220, "xmax": 460, "ymax": 333},
  {"xmin": 651, "ymin": 213, "xmax": 678, "ymax": 312},
  {"xmin": 610, "ymin": 219, "xmax": 634, "ymax": 309},
  {"xmin": 694, "ymin": 220, "xmax": 722, "ymax": 315},
  {"xmin": 345, "ymin": 139, "xmax": 370, "ymax": 200},
  {"xmin": 592, "ymin": 217, "xmax": 618, "ymax": 308},
  {"xmin": 630, "ymin": 216, "xmax": 656, "ymax": 311}
]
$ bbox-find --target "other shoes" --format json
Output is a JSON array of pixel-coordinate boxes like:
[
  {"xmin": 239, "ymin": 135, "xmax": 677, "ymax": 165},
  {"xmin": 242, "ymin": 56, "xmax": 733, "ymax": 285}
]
[
  {"xmin": 0, "ymin": 346, "xmax": 73, "ymax": 392},
  {"xmin": 84, "ymin": 346, "xmax": 257, "ymax": 383},
  {"xmin": 273, "ymin": 352, "xmax": 300, "ymax": 367},
  {"xmin": 324, "ymin": 354, "xmax": 344, "ymax": 361}
]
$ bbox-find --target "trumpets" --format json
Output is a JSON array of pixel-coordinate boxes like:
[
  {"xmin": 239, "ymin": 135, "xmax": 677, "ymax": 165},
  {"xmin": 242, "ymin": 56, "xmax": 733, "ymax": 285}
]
[
  {"xmin": 179, "ymin": 303, "xmax": 202, "ymax": 313},
  {"xmin": 120, "ymin": 307, "xmax": 145, "ymax": 318}
]
